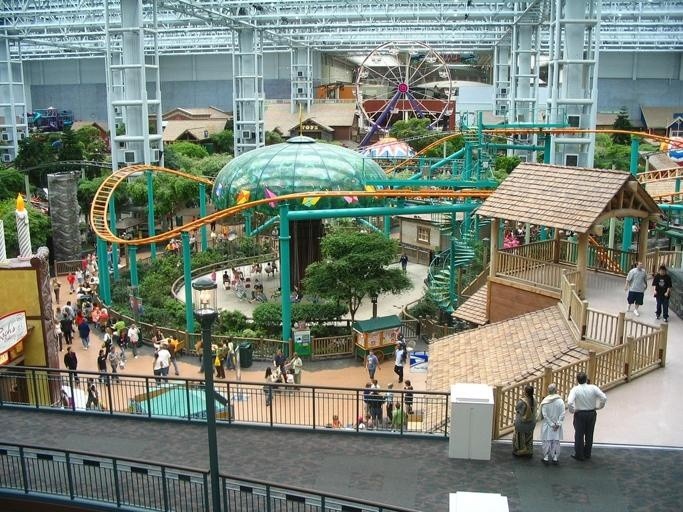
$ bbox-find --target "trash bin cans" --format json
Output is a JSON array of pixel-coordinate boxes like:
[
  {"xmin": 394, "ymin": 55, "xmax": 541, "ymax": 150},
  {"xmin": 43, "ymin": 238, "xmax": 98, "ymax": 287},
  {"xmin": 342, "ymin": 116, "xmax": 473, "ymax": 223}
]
[
  {"xmin": 176, "ymin": 215, "xmax": 183, "ymax": 226},
  {"xmin": 240, "ymin": 342, "xmax": 252, "ymax": 368}
]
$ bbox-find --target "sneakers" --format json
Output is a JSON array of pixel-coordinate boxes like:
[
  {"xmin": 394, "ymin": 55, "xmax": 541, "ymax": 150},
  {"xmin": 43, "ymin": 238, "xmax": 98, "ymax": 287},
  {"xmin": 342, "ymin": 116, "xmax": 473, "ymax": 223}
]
[
  {"xmin": 571, "ymin": 454, "xmax": 584, "ymax": 461},
  {"xmin": 552, "ymin": 459, "xmax": 558, "ymax": 465},
  {"xmin": 663, "ymin": 318, "xmax": 667, "ymax": 322},
  {"xmin": 541, "ymin": 458, "xmax": 549, "ymax": 464},
  {"xmin": 584, "ymin": 455, "xmax": 591, "ymax": 459},
  {"xmin": 656, "ymin": 316, "xmax": 660, "ymax": 319},
  {"xmin": 627, "ymin": 304, "xmax": 631, "ymax": 311},
  {"xmin": 634, "ymin": 309, "xmax": 640, "ymax": 316}
]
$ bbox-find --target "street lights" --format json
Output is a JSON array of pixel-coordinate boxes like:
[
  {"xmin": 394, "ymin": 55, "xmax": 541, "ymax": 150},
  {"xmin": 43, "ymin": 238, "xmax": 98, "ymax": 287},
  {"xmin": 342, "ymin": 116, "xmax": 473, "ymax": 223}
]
[
  {"xmin": 369, "ymin": 292, "xmax": 379, "ymax": 317},
  {"xmin": 189, "ymin": 272, "xmax": 224, "ymax": 511}
]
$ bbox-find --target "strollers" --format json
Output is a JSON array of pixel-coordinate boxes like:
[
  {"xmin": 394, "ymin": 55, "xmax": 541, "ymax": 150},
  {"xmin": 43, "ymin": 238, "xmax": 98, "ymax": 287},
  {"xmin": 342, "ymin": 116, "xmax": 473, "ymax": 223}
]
[
  {"xmin": 262, "ymin": 365, "xmax": 284, "ymax": 394},
  {"xmin": 285, "ymin": 368, "xmax": 295, "ymax": 393}
]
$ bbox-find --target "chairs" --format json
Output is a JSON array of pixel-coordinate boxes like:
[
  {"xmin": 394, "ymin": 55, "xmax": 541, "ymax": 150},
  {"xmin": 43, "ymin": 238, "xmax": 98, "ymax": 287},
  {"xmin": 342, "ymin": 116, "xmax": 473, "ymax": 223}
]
[{"xmin": 88, "ymin": 311, "xmax": 201, "ymax": 362}]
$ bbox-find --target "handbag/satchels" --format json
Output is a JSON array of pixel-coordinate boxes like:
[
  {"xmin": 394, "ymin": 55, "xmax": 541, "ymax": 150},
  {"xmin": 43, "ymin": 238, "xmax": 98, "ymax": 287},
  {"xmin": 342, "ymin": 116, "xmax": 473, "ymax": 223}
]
[{"xmin": 517, "ymin": 401, "xmax": 533, "ymax": 421}]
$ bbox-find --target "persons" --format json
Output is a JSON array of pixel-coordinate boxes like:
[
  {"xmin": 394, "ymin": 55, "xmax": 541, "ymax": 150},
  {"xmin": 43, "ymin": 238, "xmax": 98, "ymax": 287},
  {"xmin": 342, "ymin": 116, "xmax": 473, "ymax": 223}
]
[
  {"xmin": 511, "ymin": 384, "xmax": 536, "ymax": 457},
  {"xmin": 51, "ymin": 212, "xmax": 304, "ymax": 415},
  {"xmin": 649, "ymin": 266, "xmax": 672, "ymax": 322},
  {"xmin": 631, "ymin": 217, "xmax": 658, "ymax": 243},
  {"xmin": 502, "ymin": 219, "xmax": 575, "ymax": 249},
  {"xmin": 567, "ymin": 371, "xmax": 606, "ymax": 460},
  {"xmin": 398, "ymin": 252, "xmax": 408, "ymax": 273},
  {"xmin": 325, "ymin": 325, "xmax": 414, "ymax": 428},
  {"xmin": 624, "ymin": 260, "xmax": 648, "ymax": 317},
  {"xmin": 537, "ymin": 380, "xmax": 565, "ymax": 464}
]
